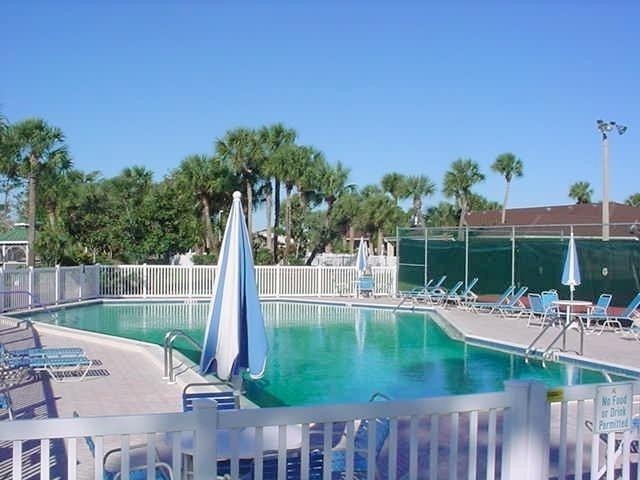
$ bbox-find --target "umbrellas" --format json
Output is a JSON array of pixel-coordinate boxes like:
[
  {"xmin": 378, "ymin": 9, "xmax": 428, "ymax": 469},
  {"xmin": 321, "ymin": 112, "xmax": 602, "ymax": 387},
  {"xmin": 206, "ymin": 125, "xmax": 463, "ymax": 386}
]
[
  {"xmin": 357, "ymin": 235, "xmax": 367, "ymax": 275},
  {"xmin": 199, "ymin": 192, "xmax": 269, "ymax": 409},
  {"xmin": 561, "ymin": 232, "xmax": 581, "ymax": 302}
]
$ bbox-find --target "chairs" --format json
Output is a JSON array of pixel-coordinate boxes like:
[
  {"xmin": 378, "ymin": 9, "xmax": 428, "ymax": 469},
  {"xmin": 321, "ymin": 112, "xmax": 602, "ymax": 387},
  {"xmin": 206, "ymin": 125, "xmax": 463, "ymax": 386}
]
[
  {"xmin": 250, "ymin": 454, "xmax": 324, "ymax": 479},
  {"xmin": 399, "ymin": 273, "xmax": 639, "ymax": 340},
  {"xmin": 72, "ymin": 411, "xmax": 173, "ymax": 479},
  {"xmin": 183, "ymin": 382, "xmax": 240, "ymax": 410},
  {"xmin": 355, "ymin": 274, "xmax": 378, "ymax": 301},
  {"xmin": 310, "ymin": 393, "xmax": 393, "ymax": 479}
]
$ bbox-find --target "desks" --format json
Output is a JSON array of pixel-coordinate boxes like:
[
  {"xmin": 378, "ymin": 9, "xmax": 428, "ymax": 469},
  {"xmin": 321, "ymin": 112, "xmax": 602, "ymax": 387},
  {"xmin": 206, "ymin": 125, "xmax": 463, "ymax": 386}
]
[{"xmin": 164, "ymin": 422, "xmax": 302, "ymax": 480}]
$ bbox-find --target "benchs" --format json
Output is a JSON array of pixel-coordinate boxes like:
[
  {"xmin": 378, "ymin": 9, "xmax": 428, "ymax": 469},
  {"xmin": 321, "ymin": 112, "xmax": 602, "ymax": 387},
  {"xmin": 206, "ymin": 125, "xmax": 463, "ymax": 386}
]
[{"xmin": 1, "ymin": 339, "xmax": 93, "ymax": 383}]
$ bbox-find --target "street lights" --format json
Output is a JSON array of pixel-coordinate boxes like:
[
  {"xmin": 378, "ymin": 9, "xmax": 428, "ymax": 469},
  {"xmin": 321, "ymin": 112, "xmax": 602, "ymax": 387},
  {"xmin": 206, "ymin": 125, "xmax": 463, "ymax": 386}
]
[{"xmin": 595, "ymin": 119, "xmax": 627, "ymax": 242}]
